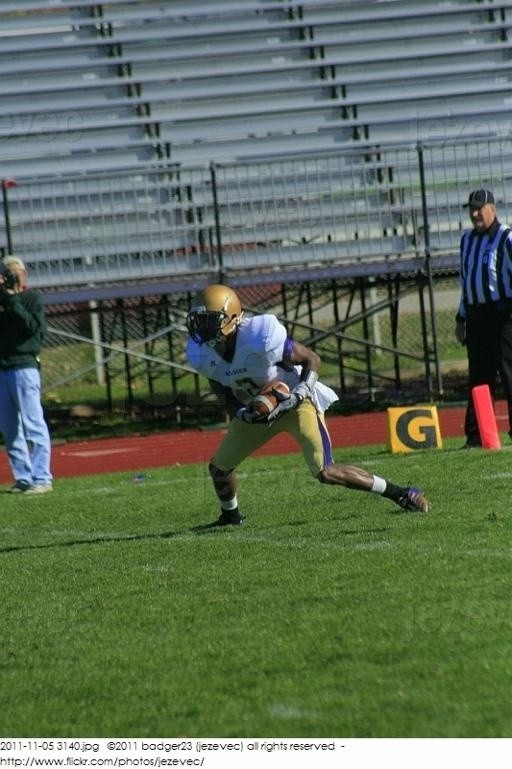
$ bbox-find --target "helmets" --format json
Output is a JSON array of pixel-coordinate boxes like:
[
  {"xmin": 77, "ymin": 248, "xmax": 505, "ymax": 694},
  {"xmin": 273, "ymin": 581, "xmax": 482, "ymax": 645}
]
[{"xmin": 197, "ymin": 284, "xmax": 241, "ymax": 325}]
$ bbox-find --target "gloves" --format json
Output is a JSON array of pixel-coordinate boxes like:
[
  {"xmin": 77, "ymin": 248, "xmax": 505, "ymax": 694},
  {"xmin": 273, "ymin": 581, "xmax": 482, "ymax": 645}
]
[
  {"xmin": 267, "ymin": 386, "xmax": 305, "ymax": 429},
  {"xmin": 233, "ymin": 405, "xmax": 268, "ymax": 425}
]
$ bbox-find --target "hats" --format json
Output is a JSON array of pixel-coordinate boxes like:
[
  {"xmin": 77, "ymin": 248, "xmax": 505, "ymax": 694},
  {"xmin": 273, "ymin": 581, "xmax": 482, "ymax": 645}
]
[{"xmin": 463, "ymin": 189, "xmax": 494, "ymax": 208}]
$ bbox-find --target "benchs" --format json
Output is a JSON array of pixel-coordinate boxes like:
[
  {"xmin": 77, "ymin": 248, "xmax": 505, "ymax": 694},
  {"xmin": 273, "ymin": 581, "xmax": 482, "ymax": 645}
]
[{"xmin": 0, "ymin": 0, "xmax": 512, "ymax": 293}]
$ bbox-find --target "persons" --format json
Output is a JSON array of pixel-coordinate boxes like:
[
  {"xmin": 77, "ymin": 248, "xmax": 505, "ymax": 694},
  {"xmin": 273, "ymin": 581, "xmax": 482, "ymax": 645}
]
[
  {"xmin": 454, "ymin": 192, "xmax": 511, "ymax": 450},
  {"xmin": 186, "ymin": 284, "xmax": 428, "ymax": 514},
  {"xmin": 2, "ymin": 258, "xmax": 54, "ymax": 495}
]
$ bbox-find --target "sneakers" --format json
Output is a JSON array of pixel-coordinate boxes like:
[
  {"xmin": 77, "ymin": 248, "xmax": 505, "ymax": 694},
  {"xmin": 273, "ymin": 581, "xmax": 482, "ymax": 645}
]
[
  {"xmin": 11, "ymin": 484, "xmax": 52, "ymax": 494},
  {"xmin": 396, "ymin": 488, "xmax": 428, "ymax": 513},
  {"xmin": 207, "ymin": 515, "xmax": 243, "ymax": 528}
]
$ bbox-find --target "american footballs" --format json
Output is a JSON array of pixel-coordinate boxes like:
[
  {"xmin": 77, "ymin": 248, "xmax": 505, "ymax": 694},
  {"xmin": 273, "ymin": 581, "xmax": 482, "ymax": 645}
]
[{"xmin": 252, "ymin": 381, "xmax": 290, "ymax": 415}]
